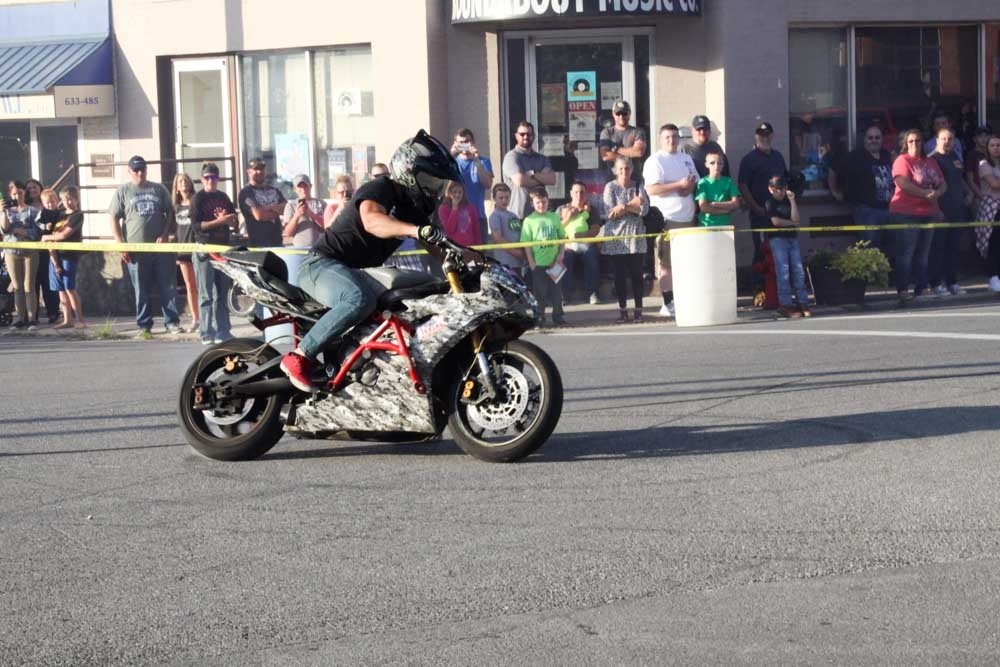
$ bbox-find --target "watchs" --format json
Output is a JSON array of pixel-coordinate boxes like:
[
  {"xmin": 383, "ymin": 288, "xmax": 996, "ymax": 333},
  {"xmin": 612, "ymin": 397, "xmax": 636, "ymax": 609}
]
[{"xmin": 530, "ymin": 169, "xmax": 537, "ymax": 179}]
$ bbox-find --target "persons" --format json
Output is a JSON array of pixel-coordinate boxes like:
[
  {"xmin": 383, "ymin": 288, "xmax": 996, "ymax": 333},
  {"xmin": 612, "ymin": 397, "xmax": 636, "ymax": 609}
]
[
  {"xmin": 788, "ymin": 70, "xmax": 987, "ymax": 181},
  {"xmin": 279, "ymin": 130, "xmax": 462, "ymax": 393},
  {"xmin": 0, "ymin": 101, "xmax": 1000, "ymax": 345}
]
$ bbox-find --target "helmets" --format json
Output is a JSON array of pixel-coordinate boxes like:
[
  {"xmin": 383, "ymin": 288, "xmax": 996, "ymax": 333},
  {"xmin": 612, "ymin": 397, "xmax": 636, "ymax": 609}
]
[{"xmin": 390, "ymin": 129, "xmax": 460, "ymax": 216}]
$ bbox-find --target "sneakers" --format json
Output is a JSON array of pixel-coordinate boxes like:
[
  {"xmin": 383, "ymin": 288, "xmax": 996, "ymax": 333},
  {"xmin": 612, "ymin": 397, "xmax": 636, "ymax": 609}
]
[{"xmin": 279, "ymin": 351, "xmax": 313, "ymax": 393}]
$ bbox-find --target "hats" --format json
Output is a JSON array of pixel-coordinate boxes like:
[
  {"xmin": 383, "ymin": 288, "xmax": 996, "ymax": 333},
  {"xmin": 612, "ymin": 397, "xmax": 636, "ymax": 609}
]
[
  {"xmin": 294, "ymin": 175, "xmax": 310, "ymax": 188},
  {"xmin": 755, "ymin": 122, "xmax": 773, "ymax": 135},
  {"xmin": 612, "ymin": 101, "xmax": 631, "ymax": 113},
  {"xmin": 248, "ymin": 157, "xmax": 266, "ymax": 169},
  {"xmin": 201, "ymin": 163, "xmax": 219, "ymax": 175},
  {"xmin": 129, "ymin": 156, "xmax": 147, "ymax": 169},
  {"xmin": 769, "ymin": 176, "xmax": 786, "ymax": 187},
  {"xmin": 692, "ymin": 115, "xmax": 710, "ymax": 129}
]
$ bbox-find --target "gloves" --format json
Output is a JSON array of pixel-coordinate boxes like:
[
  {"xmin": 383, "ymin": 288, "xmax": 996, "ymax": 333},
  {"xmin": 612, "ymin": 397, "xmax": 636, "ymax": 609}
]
[{"xmin": 418, "ymin": 224, "xmax": 443, "ymax": 243}]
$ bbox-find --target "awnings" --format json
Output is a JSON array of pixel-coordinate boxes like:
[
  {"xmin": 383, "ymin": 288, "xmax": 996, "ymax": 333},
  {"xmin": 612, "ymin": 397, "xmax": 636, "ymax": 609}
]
[{"xmin": 1, "ymin": 37, "xmax": 116, "ymax": 123}]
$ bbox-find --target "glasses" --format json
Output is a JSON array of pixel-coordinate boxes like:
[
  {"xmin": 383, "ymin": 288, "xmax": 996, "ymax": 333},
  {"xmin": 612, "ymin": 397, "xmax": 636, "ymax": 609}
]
[
  {"xmin": 202, "ymin": 175, "xmax": 218, "ymax": 181},
  {"xmin": 517, "ymin": 131, "xmax": 532, "ymax": 137}
]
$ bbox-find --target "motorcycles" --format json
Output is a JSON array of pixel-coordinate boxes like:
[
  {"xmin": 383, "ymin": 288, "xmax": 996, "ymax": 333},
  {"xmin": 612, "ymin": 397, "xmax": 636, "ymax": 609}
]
[{"xmin": 179, "ymin": 225, "xmax": 567, "ymax": 464}]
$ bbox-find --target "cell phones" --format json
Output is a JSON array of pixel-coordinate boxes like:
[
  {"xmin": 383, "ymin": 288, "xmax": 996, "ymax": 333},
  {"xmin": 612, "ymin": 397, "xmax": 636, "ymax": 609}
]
[
  {"xmin": 299, "ymin": 199, "xmax": 305, "ymax": 205},
  {"xmin": 5, "ymin": 199, "xmax": 18, "ymax": 208},
  {"xmin": 456, "ymin": 144, "xmax": 471, "ymax": 152},
  {"xmin": 346, "ymin": 192, "xmax": 352, "ymax": 201}
]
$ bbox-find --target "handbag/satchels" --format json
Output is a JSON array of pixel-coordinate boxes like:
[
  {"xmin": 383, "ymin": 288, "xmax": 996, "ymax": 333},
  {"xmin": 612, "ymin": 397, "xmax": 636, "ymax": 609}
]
[{"xmin": 643, "ymin": 206, "xmax": 665, "ymax": 241}]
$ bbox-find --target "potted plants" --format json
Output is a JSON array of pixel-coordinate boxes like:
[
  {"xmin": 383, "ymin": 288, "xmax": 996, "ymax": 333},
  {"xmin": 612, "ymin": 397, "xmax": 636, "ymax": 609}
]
[{"xmin": 804, "ymin": 240, "xmax": 893, "ymax": 307}]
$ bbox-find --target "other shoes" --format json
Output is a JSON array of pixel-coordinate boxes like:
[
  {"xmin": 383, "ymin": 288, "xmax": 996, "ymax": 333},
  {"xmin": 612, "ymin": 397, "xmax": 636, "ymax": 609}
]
[
  {"xmin": 530, "ymin": 276, "xmax": 1000, "ymax": 329},
  {"xmin": 27, "ymin": 321, "xmax": 38, "ymax": 330},
  {"xmin": 202, "ymin": 336, "xmax": 214, "ymax": 344},
  {"xmin": 54, "ymin": 323, "xmax": 73, "ymax": 329},
  {"xmin": 187, "ymin": 320, "xmax": 200, "ymax": 333},
  {"xmin": 214, "ymin": 335, "xmax": 234, "ymax": 344},
  {"xmin": 10, "ymin": 321, "xmax": 25, "ymax": 330},
  {"xmin": 75, "ymin": 323, "xmax": 86, "ymax": 328},
  {"xmin": 136, "ymin": 329, "xmax": 151, "ymax": 336},
  {"xmin": 166, "ymin": 324, "xmax": 184, "ymax": 334}
]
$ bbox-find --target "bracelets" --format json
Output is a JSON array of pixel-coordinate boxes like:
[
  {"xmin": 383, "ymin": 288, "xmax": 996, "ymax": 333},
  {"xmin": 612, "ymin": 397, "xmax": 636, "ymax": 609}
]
[
  {"xmin": 624, "ymin": 204, "xmax": 628, "ymax": 216},
  {"xmin": 612, "ymin": 145, "xmax": 618, "ymax": 153}
]
